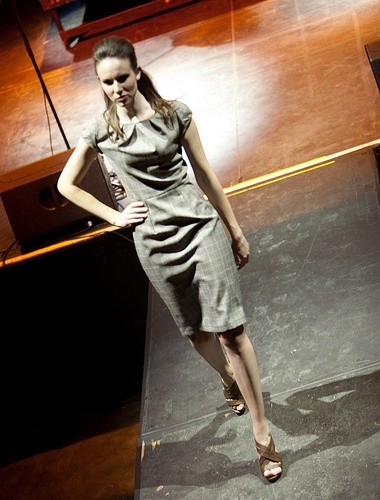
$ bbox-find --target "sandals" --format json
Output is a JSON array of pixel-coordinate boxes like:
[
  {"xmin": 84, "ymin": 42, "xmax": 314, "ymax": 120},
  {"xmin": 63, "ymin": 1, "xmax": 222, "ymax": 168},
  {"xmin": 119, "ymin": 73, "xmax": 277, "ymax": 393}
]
[
  {"xmin": 221, "ymin": 379, "xmax": 246, "ymax": 415},
  {"xmin": 253, "ymin": 432, "xmax": 283, "ymax": 482}
]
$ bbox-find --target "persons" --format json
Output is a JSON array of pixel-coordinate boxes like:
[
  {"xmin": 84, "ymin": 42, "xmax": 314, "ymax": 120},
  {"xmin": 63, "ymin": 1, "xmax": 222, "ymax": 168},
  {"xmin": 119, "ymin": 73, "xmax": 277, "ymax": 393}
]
[{"xmin": 57, "ymin": 36, "xmax": 286, "ymax": 482}]
